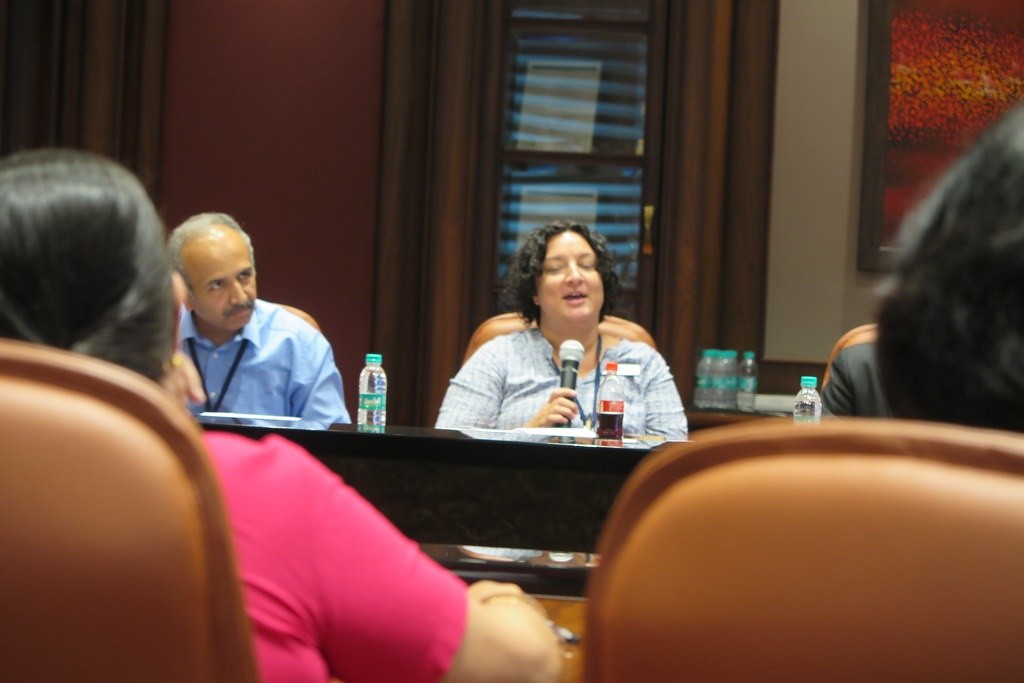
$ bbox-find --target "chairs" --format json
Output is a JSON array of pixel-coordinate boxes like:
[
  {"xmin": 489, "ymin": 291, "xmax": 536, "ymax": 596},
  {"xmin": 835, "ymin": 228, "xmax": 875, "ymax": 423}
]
[
  {"xmin": 461, "ymin": 311, "xmax": 657, "ymax": 362},
  {"xmin": 579, "ymin": 418, "xmax": 1023, "ymax": 683},
  {"xmin": 821, "ymin": 324, "xmax": 878, "ymax": 390},
  {"xmin": 0, "ymin": 335, "xmax": 260, "ymax": 683}
]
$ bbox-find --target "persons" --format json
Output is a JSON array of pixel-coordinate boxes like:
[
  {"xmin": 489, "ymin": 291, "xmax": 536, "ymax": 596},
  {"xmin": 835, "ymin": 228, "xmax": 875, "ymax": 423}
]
[
  {"xmin": 433, "ymin": 220, "xmax": 689, "ymax": 559},
  {"xmin": 814, "ymin": 102, "xmax": 1024, "ymax": 433},
  {"xmin": 0, "ymin": 147, "xmax": 561, "ymax": 683}
]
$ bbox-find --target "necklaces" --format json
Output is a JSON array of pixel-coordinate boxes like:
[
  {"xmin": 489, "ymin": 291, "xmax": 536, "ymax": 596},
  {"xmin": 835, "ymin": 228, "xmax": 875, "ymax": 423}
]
[{"xmin": 552, "ymin": 334, "xmax": 602, "ymax": 428}]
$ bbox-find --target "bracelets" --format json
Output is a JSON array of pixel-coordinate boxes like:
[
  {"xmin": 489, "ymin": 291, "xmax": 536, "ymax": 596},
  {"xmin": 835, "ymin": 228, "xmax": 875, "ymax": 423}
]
[{"xmin": 478, "ymin": 591, "xmax": 548, "ymax": 616}]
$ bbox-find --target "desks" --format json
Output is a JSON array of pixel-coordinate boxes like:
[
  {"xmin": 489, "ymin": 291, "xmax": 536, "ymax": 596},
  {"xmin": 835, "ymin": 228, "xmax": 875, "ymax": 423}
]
[
  {"xmin": 681, "ymin": 406, "xmax": 776, "ymax": 427},
  {"xmin": 190, "ymin": 413, "xmax": 656, "ymax": 601}
]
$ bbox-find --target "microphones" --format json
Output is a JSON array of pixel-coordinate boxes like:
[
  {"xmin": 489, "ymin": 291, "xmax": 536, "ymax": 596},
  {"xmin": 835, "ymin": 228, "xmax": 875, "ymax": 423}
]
[{"xmin": 554, "ymin": 338, "xmax": 585, "ymax": 428}]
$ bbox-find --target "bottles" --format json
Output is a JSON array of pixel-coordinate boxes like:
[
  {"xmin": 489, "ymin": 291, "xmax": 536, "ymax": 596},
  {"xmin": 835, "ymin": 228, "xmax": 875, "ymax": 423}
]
[
  {"xmin": 794, "ymin": 376, "xmax": 822, "ymax": 421},
  {"xmin": 358, "ymin": 353, "xmax": 387, "ymax": 434},
  {"xmin": 596, "ymin": 362, "xmax": 625, "ymax": 446},
  {"xmin": 693, "ymin": 349, "xmax": 757, "ymax": 411}
]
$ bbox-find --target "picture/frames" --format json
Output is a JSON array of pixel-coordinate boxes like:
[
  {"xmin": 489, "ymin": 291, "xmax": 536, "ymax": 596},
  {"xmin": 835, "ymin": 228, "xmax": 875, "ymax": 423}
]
[{"xmin": 855, "ymin": 1, "xmax": 1023, "ymax": 274}]
